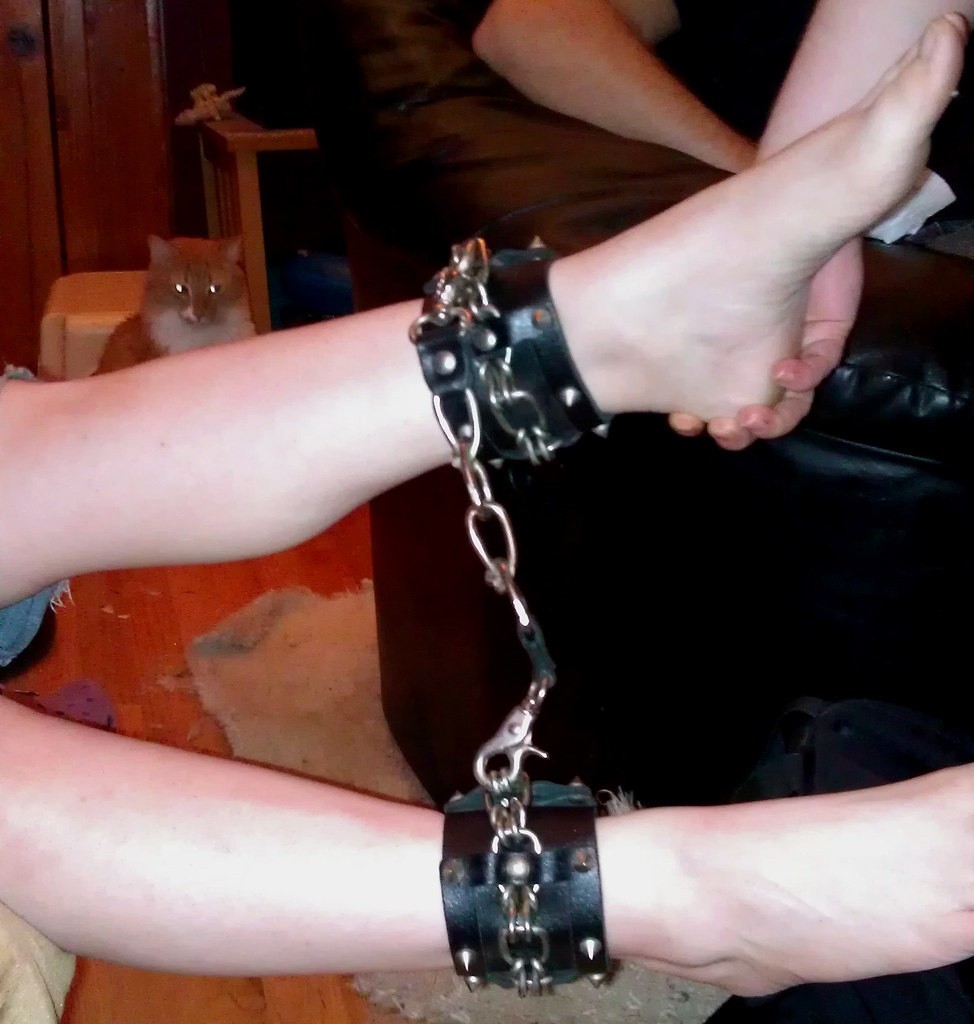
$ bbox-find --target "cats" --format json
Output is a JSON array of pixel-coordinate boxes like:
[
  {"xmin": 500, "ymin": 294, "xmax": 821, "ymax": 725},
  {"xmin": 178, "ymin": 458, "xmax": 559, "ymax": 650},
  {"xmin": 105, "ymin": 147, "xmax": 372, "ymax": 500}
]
[{"xmin": 85, "ymin": 230, "xmax": 258, "ymax": 378}]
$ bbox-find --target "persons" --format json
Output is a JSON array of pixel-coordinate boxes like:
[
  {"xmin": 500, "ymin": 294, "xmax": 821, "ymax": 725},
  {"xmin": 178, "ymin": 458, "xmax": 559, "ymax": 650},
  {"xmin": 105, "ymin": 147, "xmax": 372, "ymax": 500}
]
[
  {"xmin": 472, "ymin": 0, "xmax": 974, "ymax": 451},
  {"xmin": 1, "ymin": 7, "xmax": 974, "ymax": 1000}
]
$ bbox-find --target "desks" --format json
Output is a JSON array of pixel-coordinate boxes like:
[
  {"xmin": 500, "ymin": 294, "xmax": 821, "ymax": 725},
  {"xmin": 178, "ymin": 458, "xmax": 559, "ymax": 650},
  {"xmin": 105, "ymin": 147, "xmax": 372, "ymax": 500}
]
[{"xmin": 0, "ymin": 500, "xmax": 417, "ymax": 1024}]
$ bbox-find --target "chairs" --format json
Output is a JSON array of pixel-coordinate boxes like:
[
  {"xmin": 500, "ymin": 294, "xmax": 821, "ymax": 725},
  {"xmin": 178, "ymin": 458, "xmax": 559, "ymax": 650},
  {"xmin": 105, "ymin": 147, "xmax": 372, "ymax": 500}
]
[{"xmin": 46, "ymin": 108, "xmax": 318, "ymax": 377}]
